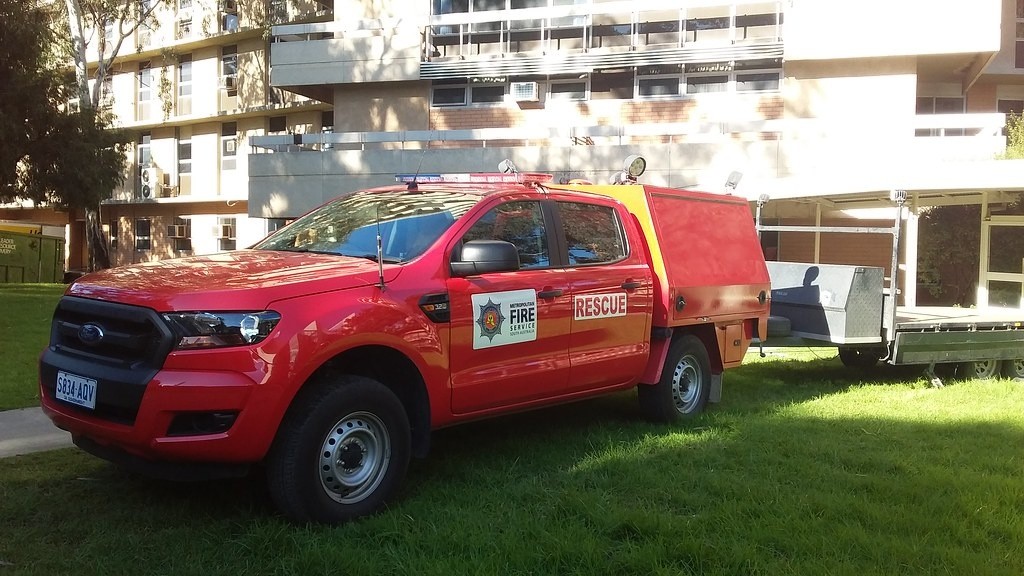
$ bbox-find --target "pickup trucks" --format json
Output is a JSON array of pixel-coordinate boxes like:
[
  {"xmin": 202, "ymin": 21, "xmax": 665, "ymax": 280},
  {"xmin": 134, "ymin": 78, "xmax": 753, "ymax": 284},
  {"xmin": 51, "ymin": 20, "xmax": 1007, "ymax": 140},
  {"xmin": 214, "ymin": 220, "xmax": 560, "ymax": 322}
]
[{"xmin": 38, "ymin": 154, "xmax": 770, "ymax": 533}]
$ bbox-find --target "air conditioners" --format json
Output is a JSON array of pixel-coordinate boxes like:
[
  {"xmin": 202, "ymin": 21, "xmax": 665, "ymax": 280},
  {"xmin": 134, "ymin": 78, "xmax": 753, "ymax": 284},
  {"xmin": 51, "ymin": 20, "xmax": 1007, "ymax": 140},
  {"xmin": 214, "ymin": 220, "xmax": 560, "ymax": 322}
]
[
  {"xmin": 510, "ymin": 81, "xmax": 540, "ymax": 102},
  {"xmin": 210, "ymin": 223, "xmax": 231, "ymax": 238},
  {"xmin": 140, "ymin": 167, "xmax": 164, "ymax": 199},
  {"xmin": 223, "ymin": 14, "xmax": 238, "ymax": 30},
  {"xmin": 226, "ymin": 77, "xmax": 235, "ymax": 89},
  {"xmin": 167, "ymin": 225, "xmax": 187, "ymax": 239}
]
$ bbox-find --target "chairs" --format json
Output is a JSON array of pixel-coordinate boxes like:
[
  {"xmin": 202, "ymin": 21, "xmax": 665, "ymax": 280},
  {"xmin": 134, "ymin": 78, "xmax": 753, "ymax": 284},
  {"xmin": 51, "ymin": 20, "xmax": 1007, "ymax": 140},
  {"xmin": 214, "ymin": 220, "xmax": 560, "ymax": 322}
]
[
  {"xmin": 460, "ymin": 208, "xmax": 497, "ymax": 243},
  {"xmin": 504, "ymin": 215, "xmax": 545, "ymax": 265}
]
